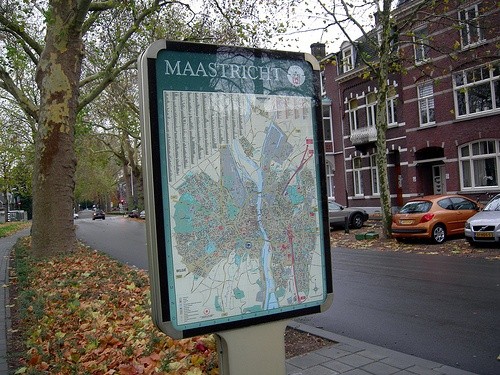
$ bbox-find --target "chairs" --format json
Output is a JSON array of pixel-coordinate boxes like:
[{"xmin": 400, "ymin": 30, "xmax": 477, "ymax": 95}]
[{"xmin": 442, "ymin": 204, "xmax": 452, "ymax": 210}]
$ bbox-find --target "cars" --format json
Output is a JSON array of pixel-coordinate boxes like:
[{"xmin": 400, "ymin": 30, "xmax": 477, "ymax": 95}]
[
  {"xmin": 129, "ymin": 210, "xmax": 139, "ymax": 218},
  {"xmin": 139, "ymin": 210, "xmax": 146, "ymax": 219},
  {"xmin": 328, "ymin": 201, "xmax": 369, "ymax": 229},
  {"xmin": 92, "ymin": 210, "xmax": 105, "ymax": 220},
  {"xmin": 462, "ymin": 194, "xmax": 500, "ymax": 246},
  {"xmin": 391, "ymin": 194, "xmax": 486, "ymax": 244}
]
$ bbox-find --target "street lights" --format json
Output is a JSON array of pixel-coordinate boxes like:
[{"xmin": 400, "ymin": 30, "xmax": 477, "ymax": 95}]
[{"xmin": 127, "ymin": 166, "xmax": 134, "ymax": 197}]
